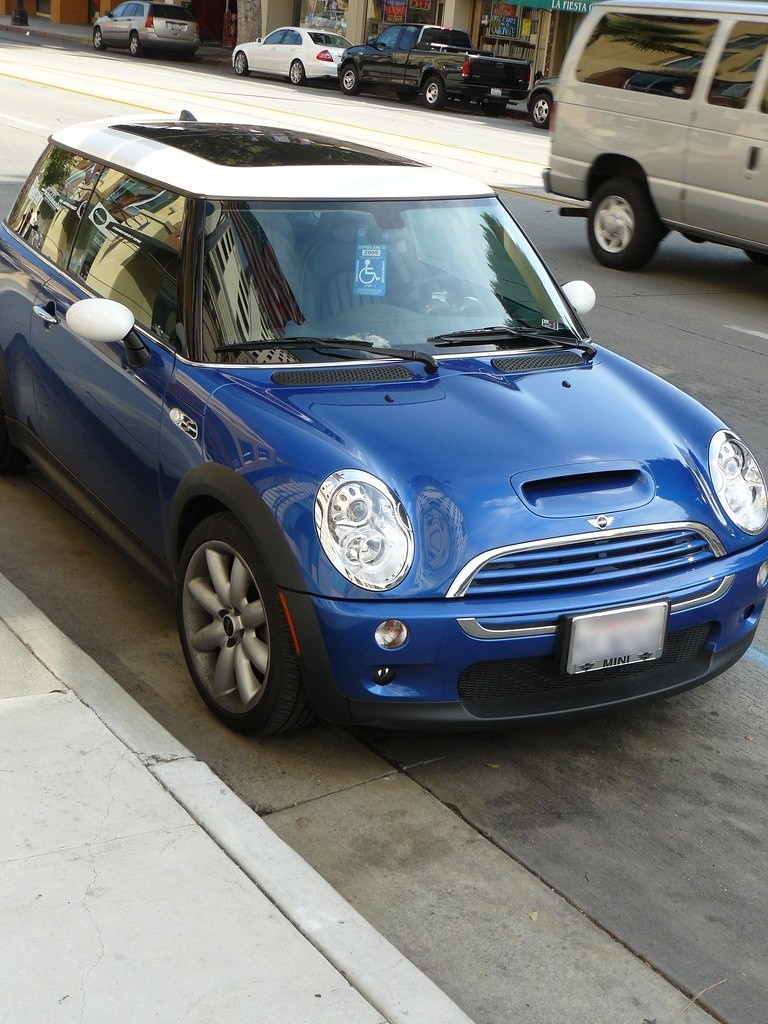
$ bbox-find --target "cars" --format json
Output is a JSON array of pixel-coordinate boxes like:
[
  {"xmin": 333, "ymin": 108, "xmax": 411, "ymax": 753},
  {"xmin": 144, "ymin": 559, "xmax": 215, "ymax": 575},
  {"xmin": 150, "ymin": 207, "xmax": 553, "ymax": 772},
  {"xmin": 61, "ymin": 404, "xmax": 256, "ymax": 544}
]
[
  {"xmin": 0, "ymin": 109, "xmax": 768, "ymax": 739},
  {"xmin": 231, "ymin": 25, "xmax": 356, "ymax": 86},
  {"xmin": 527, "ymin": 75, "xmax": 560, "ymax": 132},
  {"xmin": 91, "ymin": 0, "xmax": 200, "ymax": 56}
]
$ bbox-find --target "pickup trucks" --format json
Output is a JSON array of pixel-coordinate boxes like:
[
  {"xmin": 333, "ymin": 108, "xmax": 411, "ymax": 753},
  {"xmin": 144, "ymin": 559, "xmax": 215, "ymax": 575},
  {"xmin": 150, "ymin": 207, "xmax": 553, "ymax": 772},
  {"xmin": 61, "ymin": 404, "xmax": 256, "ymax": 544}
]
[{"xmin": 336, "ymin": 22, "xmax": 532, "ymax": 116}]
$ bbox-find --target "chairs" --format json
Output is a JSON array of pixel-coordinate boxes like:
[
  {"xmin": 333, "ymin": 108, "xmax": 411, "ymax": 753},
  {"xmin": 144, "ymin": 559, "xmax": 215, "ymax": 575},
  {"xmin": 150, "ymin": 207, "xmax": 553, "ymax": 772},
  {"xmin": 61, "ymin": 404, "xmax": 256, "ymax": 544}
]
[{"xmin": 292, "ymin": 211, "xmax": 422, "ymax": 320}]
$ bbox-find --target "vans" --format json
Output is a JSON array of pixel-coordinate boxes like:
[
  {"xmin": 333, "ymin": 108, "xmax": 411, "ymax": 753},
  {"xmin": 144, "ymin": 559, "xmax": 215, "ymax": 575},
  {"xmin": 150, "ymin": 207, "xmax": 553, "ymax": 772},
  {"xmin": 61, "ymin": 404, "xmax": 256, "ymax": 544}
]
[{"xmin": 539, "ymin": 0, "xmax": 768, "ymax": 273}]
